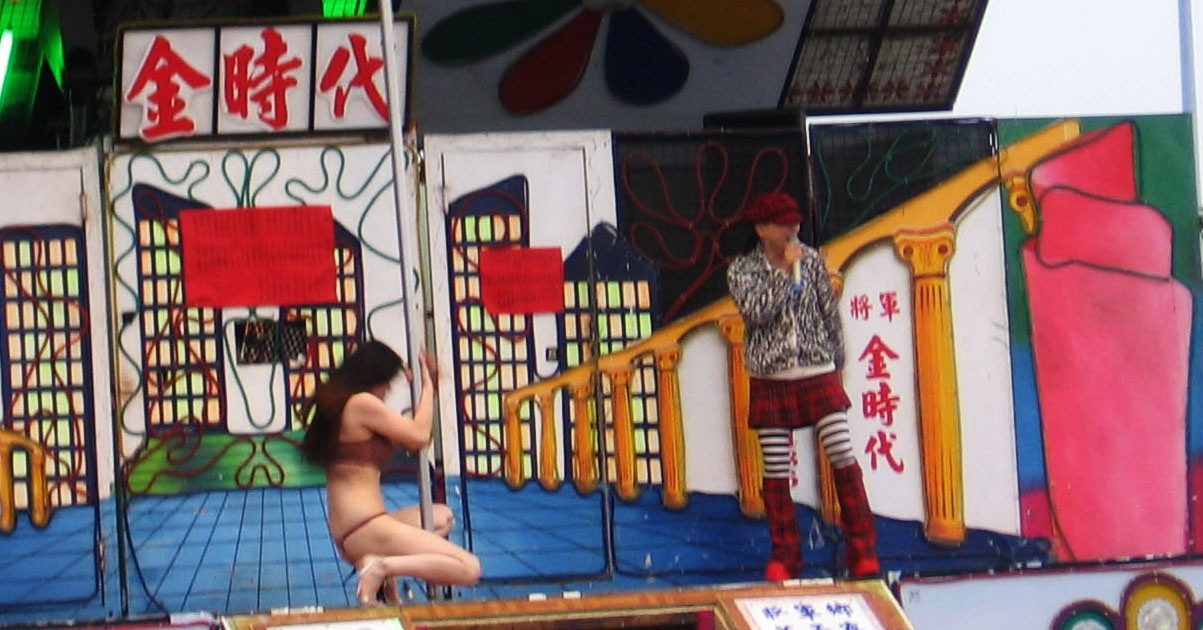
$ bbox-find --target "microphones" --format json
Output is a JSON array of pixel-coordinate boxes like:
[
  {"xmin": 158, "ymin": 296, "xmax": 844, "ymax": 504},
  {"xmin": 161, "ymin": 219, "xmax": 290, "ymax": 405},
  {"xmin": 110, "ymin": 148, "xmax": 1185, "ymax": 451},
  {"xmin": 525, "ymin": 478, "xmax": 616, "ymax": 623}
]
[{"xmin": 789, "ymin": 233, "xmax": 807, "ymax": 291}]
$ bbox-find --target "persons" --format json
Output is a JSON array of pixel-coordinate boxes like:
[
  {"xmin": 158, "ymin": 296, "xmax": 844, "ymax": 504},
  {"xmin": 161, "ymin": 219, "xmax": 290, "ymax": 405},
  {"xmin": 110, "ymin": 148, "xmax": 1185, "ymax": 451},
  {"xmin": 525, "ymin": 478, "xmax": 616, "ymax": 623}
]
[
  {"xmin": 299, "ymin": 341, "xmax": 481, "ymax": 608},
  {"xmin": 727, "ymin": 191, "xmax": 882, "ymax": 581}
]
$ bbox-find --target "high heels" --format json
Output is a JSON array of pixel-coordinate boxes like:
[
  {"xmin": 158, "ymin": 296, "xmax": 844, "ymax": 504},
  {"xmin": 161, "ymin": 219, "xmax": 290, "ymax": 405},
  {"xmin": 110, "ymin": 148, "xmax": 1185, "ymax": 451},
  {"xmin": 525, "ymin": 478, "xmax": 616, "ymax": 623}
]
[
  {"xmin": 342, "ymin": 555, "xmax": 385, "ymax": 609},
  {"xmin": 377, "ymin": 576, "xmax": 402, "ymax": 607}
]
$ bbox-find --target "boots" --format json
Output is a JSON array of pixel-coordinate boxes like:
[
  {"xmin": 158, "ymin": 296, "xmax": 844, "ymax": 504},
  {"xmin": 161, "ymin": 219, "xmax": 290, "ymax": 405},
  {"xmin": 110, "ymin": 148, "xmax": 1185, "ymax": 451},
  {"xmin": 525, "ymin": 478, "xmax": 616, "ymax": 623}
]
[
  {"xmin": 832, "ymin": 461, "xmax": 880, "ymax": 581},
  {"xmin": 761, "ymin": 478, "xmax": 803, "ymax": 581}
]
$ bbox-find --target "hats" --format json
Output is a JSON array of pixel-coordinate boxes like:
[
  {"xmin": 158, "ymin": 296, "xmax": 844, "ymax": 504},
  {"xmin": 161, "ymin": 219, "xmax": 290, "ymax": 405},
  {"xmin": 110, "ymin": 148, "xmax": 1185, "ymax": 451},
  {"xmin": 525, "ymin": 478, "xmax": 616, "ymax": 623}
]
[{"xmin": 745, "ymin": 193, "xmax": 802, "ymax": 226}]
